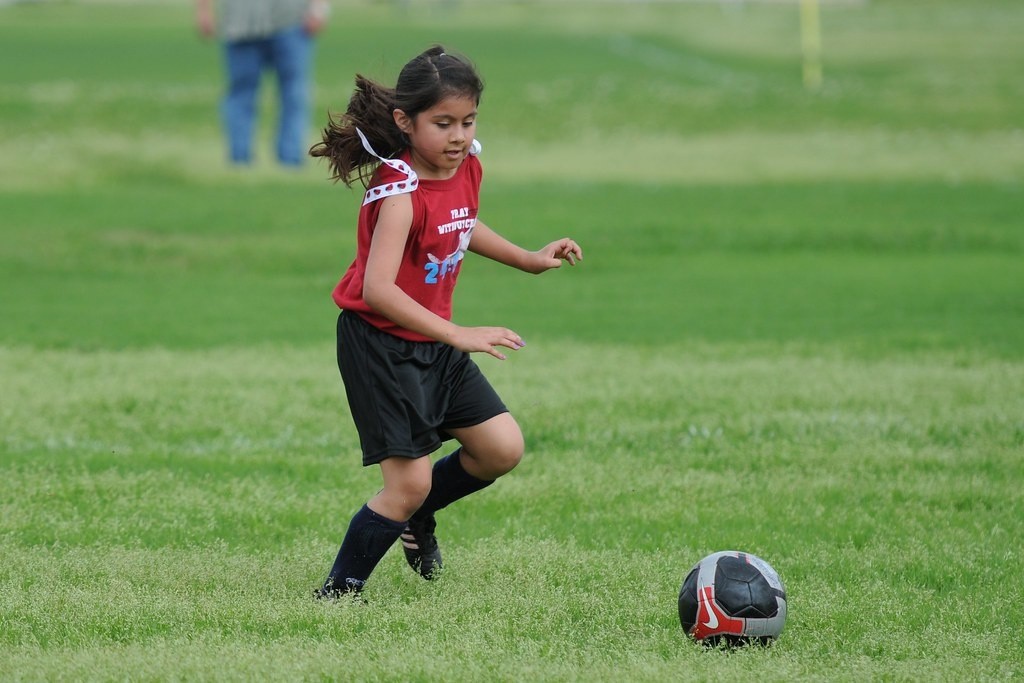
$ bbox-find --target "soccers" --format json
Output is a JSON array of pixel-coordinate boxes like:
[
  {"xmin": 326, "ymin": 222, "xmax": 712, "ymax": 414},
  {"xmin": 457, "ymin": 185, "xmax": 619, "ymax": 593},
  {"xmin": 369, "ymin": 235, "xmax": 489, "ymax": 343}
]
[{"xmin": 678, "ymin": 550, "xmax": 788, "ymax": 655}]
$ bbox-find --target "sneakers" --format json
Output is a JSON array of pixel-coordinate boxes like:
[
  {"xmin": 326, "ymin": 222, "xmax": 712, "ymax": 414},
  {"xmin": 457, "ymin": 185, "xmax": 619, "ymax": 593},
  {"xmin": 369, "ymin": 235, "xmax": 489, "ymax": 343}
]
[{"xmin": 399, "ymin": 515, "xmax": 443, "ymax": 581}]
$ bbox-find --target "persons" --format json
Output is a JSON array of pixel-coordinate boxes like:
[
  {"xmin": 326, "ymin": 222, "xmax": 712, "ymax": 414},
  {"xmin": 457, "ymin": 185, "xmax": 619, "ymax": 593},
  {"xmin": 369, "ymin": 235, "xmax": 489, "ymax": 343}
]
[
  {"xmin": 307, "ymin": 44, "xmax": 584, "ymax": 605},
  {"xmin": 194, "ymin": 0, "xmax": 329, "ymax": 166}
]
